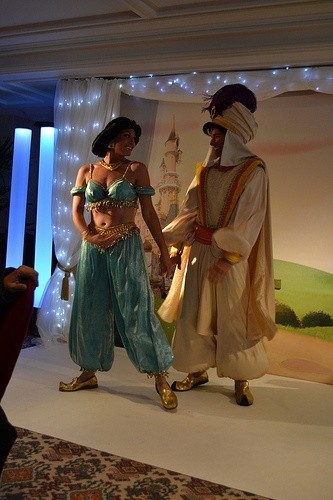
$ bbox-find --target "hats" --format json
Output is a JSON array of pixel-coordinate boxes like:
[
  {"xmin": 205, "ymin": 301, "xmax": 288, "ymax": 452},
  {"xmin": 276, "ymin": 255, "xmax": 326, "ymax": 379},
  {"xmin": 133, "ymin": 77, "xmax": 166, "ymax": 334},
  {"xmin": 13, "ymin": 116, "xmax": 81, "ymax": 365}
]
[
  {"xmin": 201, "ymin": 101, "xmax": 258, "ymax": 169},
  {"xmin": 92, "ymin": 117, "xmax": 142, "ymax": 156}
]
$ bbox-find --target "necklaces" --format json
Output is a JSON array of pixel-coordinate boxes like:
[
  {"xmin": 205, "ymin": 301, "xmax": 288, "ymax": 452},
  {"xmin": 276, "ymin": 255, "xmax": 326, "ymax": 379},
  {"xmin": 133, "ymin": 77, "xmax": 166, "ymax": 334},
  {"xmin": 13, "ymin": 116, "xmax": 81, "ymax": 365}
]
[{"xmin": 100, "ymin": 159, "xmax": 128, "ymax": 171}]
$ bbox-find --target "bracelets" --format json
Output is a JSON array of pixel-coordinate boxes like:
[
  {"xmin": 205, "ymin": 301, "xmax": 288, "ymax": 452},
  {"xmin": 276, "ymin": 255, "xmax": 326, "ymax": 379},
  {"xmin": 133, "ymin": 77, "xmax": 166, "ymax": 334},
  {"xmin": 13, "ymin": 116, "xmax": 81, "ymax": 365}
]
[{"xmin": 82, "ymin": 229, "xmax": 92, "ymax": 241}]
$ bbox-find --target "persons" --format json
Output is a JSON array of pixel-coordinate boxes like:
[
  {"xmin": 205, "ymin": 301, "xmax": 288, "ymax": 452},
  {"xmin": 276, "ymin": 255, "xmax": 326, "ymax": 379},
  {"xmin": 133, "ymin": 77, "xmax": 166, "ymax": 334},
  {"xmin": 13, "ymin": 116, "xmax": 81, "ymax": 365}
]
[
  {"xmin": 59, "ymin": 116, "xmax": 178, "ymax": 410},
  {"xmin": 157, "ymin": 83, "xmax": 278, "ymax": 406},
  {"xmin": 0, "ymin": 265, "xmax": 38, "ymax": 475}
]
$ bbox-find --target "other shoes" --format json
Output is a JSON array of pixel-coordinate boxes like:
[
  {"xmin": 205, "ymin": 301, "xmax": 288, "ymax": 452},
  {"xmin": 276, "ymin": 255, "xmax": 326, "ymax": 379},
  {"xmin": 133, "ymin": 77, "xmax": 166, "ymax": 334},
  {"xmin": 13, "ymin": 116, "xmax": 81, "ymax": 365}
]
[
  {"xmin": 171, "ymin": 372, "xmax": 209, "ymax": 390},
  {"xmin": 59, "ymin": 375, "xmax": 100, "ymax": 392},
  {"xmin": 233, "ymin": 380, "xmax": 255, "ymax": 406},
  {"xmin": 153, "ymin": 381, "xmax": 178, "ymax": 410}
]
